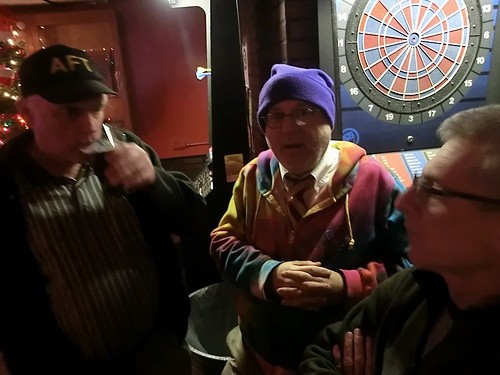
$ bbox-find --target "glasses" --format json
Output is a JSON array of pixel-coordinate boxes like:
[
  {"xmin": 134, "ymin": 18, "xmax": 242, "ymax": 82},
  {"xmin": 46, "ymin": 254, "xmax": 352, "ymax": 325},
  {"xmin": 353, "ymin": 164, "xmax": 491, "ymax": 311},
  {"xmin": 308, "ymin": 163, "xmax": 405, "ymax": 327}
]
[
  {"xmin": 409, "ymin": 172, "xmax": 499, "ymax": 216},
  {"xmin": 260, "ymin": 105, "xmax": 321, "ymax": 130}
]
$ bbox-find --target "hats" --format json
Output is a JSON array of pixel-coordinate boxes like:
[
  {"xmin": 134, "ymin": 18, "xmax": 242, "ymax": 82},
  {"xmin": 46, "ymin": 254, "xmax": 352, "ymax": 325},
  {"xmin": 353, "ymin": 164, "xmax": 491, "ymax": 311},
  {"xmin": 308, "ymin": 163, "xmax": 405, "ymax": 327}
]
[
  {"xmin": 18, "ymin": 43, "xmax": 118, "ymax": 104},
  {"xmin": 257, "ymin": 63, "xmax": 335, "ymax": 133}
]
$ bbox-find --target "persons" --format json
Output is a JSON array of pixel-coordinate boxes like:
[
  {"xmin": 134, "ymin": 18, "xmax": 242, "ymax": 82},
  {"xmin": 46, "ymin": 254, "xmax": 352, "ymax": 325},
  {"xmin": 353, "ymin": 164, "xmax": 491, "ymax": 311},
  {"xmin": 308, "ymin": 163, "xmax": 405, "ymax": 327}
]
[
  {"xmin": 210, "ymin": 64, "xmax": 410, "ymax": 375},
  {"xmin": 300, "ymin": 104, "xmax": 500, "ymax": 375},
  {"xmin": 0, "ymin": 45, "xmax": 210, "ymax": 375}
]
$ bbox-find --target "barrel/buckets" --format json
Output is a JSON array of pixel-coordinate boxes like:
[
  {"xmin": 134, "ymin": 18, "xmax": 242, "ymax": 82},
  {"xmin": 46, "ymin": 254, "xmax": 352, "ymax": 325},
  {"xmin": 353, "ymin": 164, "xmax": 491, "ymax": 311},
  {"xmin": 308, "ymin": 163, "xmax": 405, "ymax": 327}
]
[{"xmin": 182, "ymin": 282, "xmax": 236, "ymax": 375}]
